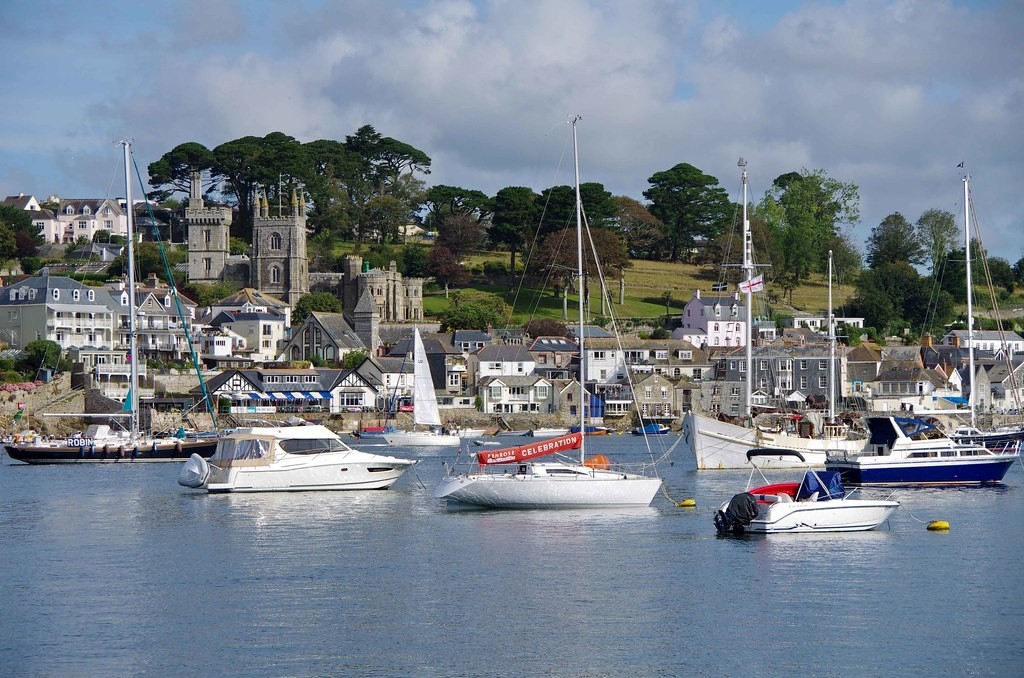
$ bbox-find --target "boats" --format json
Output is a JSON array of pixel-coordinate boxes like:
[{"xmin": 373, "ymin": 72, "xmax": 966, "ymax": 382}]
[
  {"xmin": 493, "ymin": 429, "xmax": 530, "ymax": 436},
  {"xmin": 570, "ymin": 425, "xmax": 617, "ymax": 435},
  {"xmin": 907, "ymin": 162, "xmax": 1024, "ymax": 452},
  {"xmin": 239, "ymin": 413, "xmax": 316, "ymax": 429},
  {"xmin": 351, "ymin": 423, "xmax": 401, "ymax": 440},
  {"xmin": 435, "ymin": 113, "xmax": 665, "ymax": 509},
  {"xmin": 823, "ymin": 399, "xmax": 1023, "ymax": 489},
  {"xmin": 533, "ymin": 428, "xmax": 569, "ymax": 437},
  {"xmin": 4, "ymin": 138, "xmax": 230, "ymax": 466},
  {"xmin": 1, "ymin": 400, "xmax": 37, "ymax": 444},
  {"xmin": 633, "ymin": 423, "xmax": 671, "ymax": 434},
  {"xmin": 679, "ymin": 169, "xmax": 873, "ymax": 472},
  {"xmin": 713, "ymin": 447, "xmax": 901, "ymax": 536},
  {"xmin": 447, "ymin": 426, "xmax": 487, "ymax": 438},
  {"xmin": 177, "ymin": 425, "xmax": 424, "ymax": 493}
]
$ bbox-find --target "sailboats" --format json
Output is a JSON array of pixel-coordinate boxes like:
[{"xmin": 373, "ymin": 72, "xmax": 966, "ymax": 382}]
[{"xmin": 383, "ymin": 321, "xmax": 460, "ymax": 447}]
